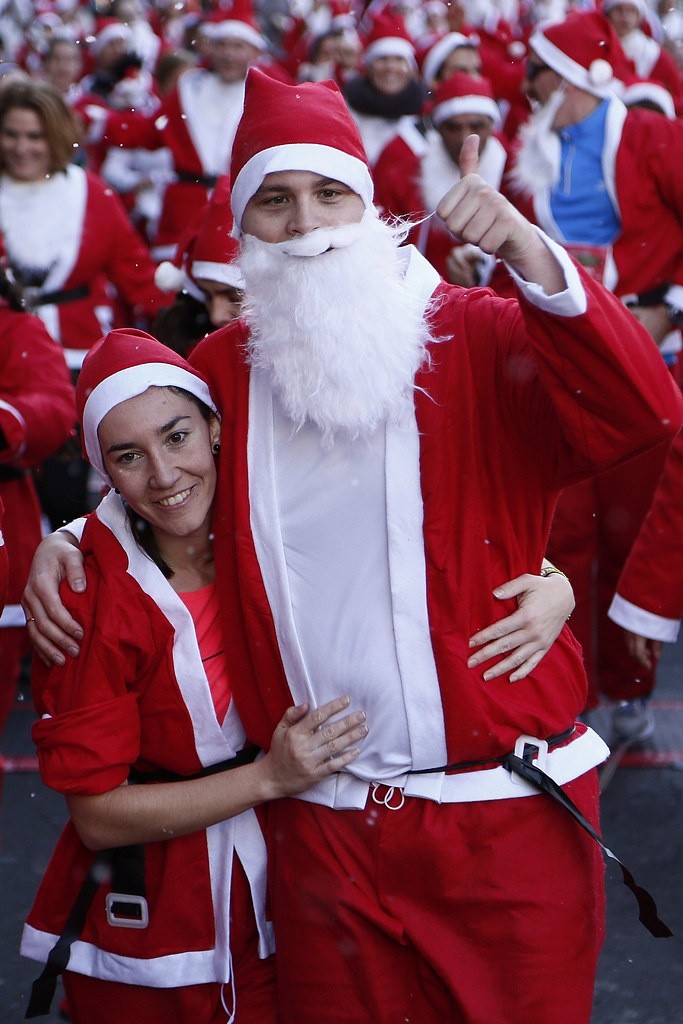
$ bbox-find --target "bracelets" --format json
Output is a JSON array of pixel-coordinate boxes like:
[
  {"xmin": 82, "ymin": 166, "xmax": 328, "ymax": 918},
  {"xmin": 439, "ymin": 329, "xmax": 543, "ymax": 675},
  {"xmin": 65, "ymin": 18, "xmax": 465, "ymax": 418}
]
[{"xmin": 540, "ymin": 567, "xmax": 571, "ymax": 620}]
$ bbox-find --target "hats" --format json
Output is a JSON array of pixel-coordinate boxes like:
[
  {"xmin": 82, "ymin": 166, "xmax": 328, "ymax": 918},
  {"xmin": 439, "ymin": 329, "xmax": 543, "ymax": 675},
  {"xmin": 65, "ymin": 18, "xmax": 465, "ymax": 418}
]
[
  {"xmin": 413, "ymin": 33, "xmax": 474, "ymax": 92},
  {"xmin": 432, "ymin": 71, "xmax": 500, "ymax": 129},
  {"xmin": 529, "ymin": 8, "xmax": 621, "ymax": 100},
  {"xmin": 155, "ymin": 171, "xmax": 244, "ymax": 293},
  {"xmin": 619, "ymin": 74, "xmax": 675, "ymax": 120},
  {"xmin": 74, "ymin": 327, "xmax": 221, "ymax": 489},
  {"xmin": 23, "ymin": 0, "xmax": 647, "ymax": 69},
  {"xmin": 231, "ymin": 66, "xmax": 373, "ymax": 236}
]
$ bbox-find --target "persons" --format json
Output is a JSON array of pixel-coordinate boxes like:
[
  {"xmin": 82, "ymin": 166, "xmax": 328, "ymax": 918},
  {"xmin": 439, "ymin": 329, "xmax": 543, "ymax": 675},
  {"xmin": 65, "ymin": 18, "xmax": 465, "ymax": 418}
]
[
  {"xmin": 22, "ymin": 67, "xmax": 683, "ymax": 1024},
  {"xmin": 16, "ymin": 329, "xmax": 576, "ymax": 1024},
  {"xmin": 0, "ymin": 0, "xmax": 683, "ymax": 743}
]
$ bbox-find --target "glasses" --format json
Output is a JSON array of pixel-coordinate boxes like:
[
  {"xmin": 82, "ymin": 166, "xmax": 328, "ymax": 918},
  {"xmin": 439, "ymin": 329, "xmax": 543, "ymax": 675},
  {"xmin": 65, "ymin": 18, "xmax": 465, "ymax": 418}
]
[
  {"xmin": 442, "ymin": 120, "xmax": 490, "ymax": 132},
  {"xmin": 525, "ymin": 59, "xmax": 550, "ymax": 80}
]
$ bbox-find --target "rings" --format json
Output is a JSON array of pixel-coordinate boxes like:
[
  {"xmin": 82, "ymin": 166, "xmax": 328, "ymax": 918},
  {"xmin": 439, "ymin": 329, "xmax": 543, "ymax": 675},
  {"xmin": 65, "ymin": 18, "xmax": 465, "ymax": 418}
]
[{"xmin": 26, "ymin": 617, "xmax": 35, "ymax": 626}]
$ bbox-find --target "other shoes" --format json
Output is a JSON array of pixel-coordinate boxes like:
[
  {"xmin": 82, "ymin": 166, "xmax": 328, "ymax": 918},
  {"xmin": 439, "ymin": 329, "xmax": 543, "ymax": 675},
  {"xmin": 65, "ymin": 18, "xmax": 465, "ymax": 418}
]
[{"xmin": 612, "ymin": 696, "xmax": 653, "ymax": 738}]
